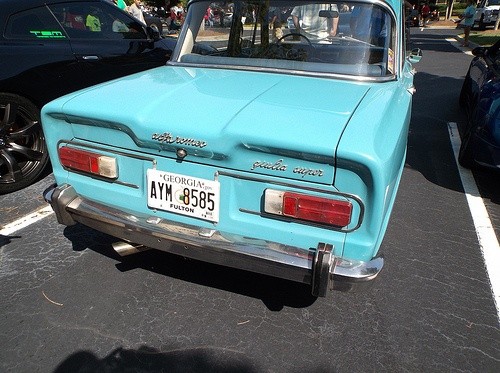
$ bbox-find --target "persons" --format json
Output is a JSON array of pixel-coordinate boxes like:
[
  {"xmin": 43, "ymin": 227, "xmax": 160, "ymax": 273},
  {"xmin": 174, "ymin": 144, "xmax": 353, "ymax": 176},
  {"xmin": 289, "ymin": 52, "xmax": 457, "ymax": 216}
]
[
  {"xmin": 66, "ymin": 0, "xmax": 152, "ymax": 33},
  {"xmin": 460, "ymin": 0, "xmax": 475, "ymax": 46},
  {"xmin": 271, "ymin": 3, "xmax": 349, "ymax": 45},
  {"xmin": 412, "ymin": 4, "xmax": 439, "ymax": 27},
  {"xmin": 169, "ymin": 1, "xmax": 214, "ymax": 31}
]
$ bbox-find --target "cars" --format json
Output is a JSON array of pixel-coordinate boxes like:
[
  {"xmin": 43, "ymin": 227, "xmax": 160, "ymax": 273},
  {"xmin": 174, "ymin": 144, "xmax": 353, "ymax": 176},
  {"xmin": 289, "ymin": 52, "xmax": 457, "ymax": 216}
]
[
  {"xmin": 41, "ymin": 0, "xmax": 423, "ymax": 298},
  {"xmin": 142, "ymin": 12, "xmax": 163, "ymax": 33},
  {"xmin": 458, "ymin": 38, "xmax": 500, "ymax": 179},
  {"xmin": 0, "ymin": 0, "xmax": 220, "ymax": 195},
  {"xmin": 475, "ymin": 0, "xmax": 500, "ymax": 31}
]
